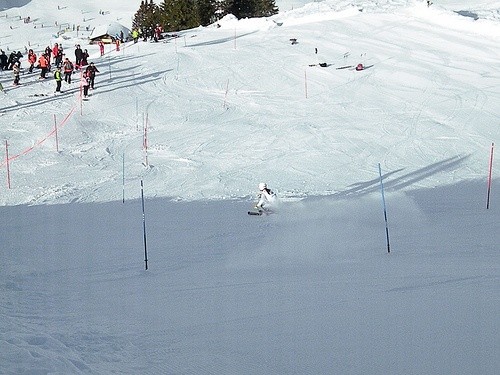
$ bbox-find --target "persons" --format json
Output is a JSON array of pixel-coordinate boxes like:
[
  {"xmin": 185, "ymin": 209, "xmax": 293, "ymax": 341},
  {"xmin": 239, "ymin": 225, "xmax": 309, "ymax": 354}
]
[
  {"xmin": 0, "ymin": 0, "xmax": 165, "ymax": 100},
  {"xmin": 247, "ymin": 182, "xmax": 279, "ymax": 217}
]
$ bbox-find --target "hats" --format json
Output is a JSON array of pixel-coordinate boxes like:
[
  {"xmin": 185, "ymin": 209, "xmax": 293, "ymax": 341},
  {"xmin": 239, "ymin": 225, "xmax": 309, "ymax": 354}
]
[
  {"xmin": 90, "ymin": 62, "xmax": 95, "ymax": 64},
  {"xmin": 259, "ymin": 183, "xmax": 267, "ymax": 190}
]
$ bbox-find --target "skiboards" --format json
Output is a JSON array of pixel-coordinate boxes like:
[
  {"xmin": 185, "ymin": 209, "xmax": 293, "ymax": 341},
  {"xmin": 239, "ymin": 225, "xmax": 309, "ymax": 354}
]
[
  {"xmin": 308, "ymin": 63, "xmax": 374, "ymax": 71},
  {"xmin": 248, "ymin": 210, "xmax": 272, "ymax": 215}
]
